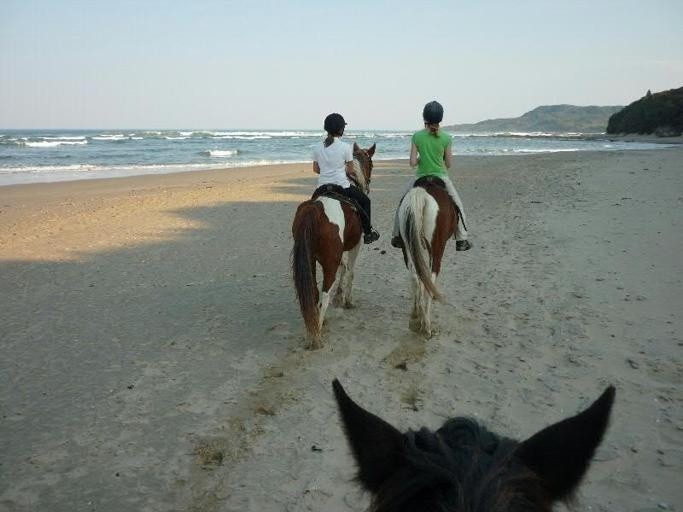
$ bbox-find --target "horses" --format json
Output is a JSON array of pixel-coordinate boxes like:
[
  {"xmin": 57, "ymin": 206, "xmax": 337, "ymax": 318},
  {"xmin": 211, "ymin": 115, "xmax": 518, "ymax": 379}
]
[
  {"xmin": 288, "ymin": 143, "xmax": 376, "ymax": 351},
  {"xmin": 398, "ymin": 183, "xmax": 458, "ymax": 339},
  {"xmin": 331, "ymin": 377, "xmax": 616, "ymax": 512}
]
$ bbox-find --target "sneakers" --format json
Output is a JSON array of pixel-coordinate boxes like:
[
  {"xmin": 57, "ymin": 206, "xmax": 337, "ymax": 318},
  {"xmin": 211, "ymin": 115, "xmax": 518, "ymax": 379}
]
[
  {"xmin": 390, "ymin": 236, "xmax": 403, "ymax": 248},
  {"xmin": 455, "ymin": 238, "xmax": 473, "ymax": 252},
  {"xmin": 363, "ymin": 230, "xmax": 380, "ymax": 246}
]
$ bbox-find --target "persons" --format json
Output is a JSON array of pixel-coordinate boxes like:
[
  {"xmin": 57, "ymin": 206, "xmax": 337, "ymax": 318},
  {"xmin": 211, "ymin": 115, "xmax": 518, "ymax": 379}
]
[
  {"xmin": 391, "ymin": 101, "xmax": 472, "ymax": 252},
  {"xmin": 309, "ymin": 112, "xmax": 381, "ymax": 245}
]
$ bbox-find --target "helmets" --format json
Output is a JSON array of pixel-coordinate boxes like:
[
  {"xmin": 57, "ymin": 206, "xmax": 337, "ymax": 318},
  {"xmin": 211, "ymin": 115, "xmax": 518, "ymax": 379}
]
[
  {"xmin": 324, "ymin": 112, "xmax": 348, "ymax": 132},
  {"xmin": 422, "ymin": 100, "xmax": 444, "ymax": 124}
]
